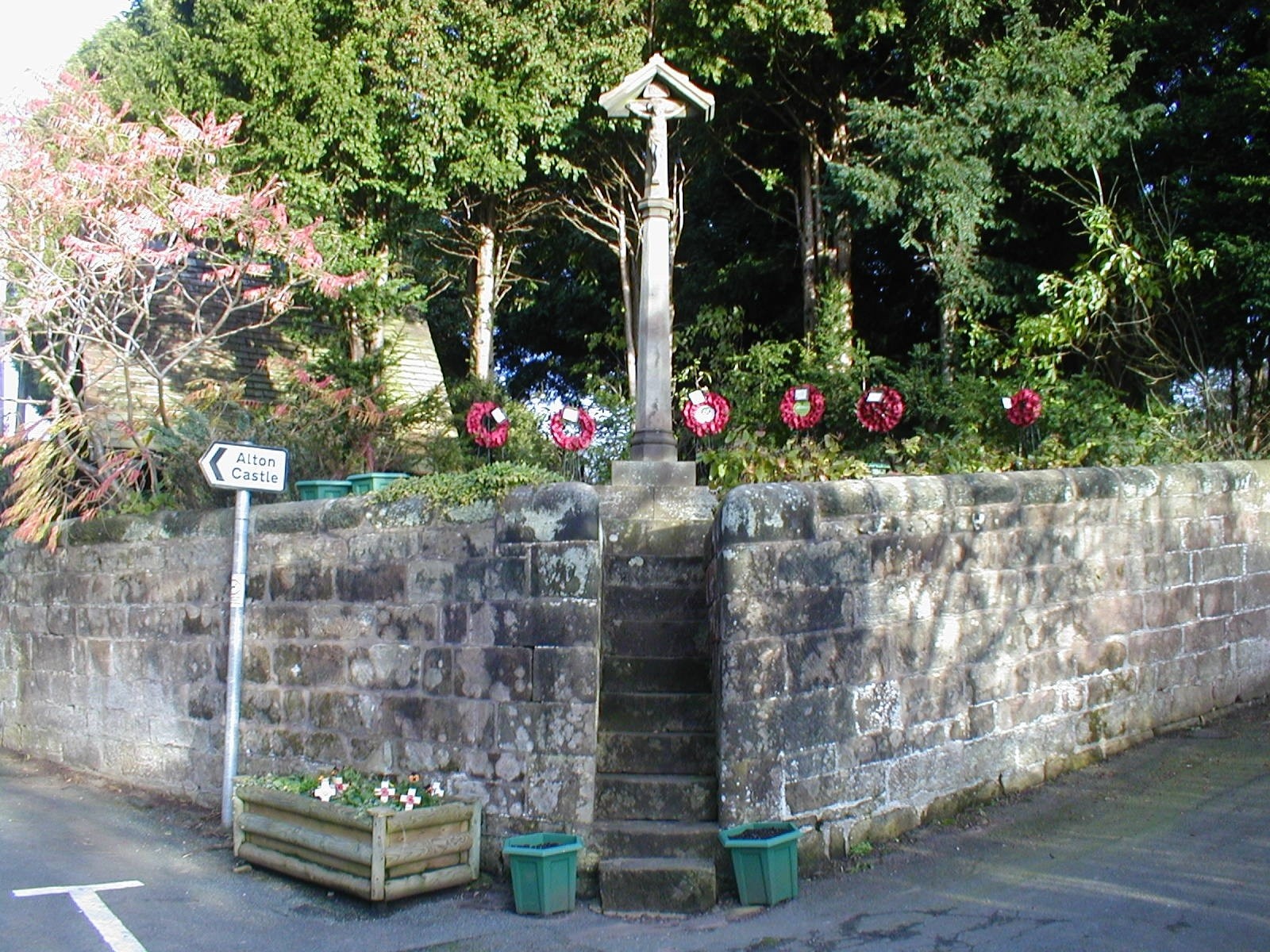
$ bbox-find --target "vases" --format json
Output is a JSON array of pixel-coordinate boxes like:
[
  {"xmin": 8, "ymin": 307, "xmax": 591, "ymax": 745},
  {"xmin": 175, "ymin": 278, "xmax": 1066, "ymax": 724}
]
[
  {"xmin": 293, "ymin": 479, "xmax": 352, "ymax": 504},
  {"xmin": 348, "ymin": 472, "xmax": 411, "ymax": 497},
  {"xmin": 721, "ymin": 820, "xmax": 804, "ymax": 907},
  {"xmin": 229, "ymin": 775, "xmax": 481, "ymax": 911},
  {"xmin": 502, "ymin": 831, "xmax": 585, "ymax": 915}
]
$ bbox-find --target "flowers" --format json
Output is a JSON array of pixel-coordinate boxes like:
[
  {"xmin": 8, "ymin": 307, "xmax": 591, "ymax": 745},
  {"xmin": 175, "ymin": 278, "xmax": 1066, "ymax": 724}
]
[
  {"xmin": 550, "ymin": 406, "xmax": 595, "ymax": 450},
  {"xmin": 1008, "ymin": 391, "xmax": 1041, "ymax": 426},
  {"xmin": 858, "ymin": 387, "xmax": 903, "ymax": 431},
  {"xmin": 255, "ymin": 765, "xmax": 446, "ymax": 811},
  {"xmin": 780, "ymin": 385, "xmax": 825, "ymax": 431},
  {"xmin": 465, "ymin": 401, "xmax": 509, "ymax": 447},
  {"xmin": 682, "ymin": 393, "xmax": 729, "ymax": 435}
]
